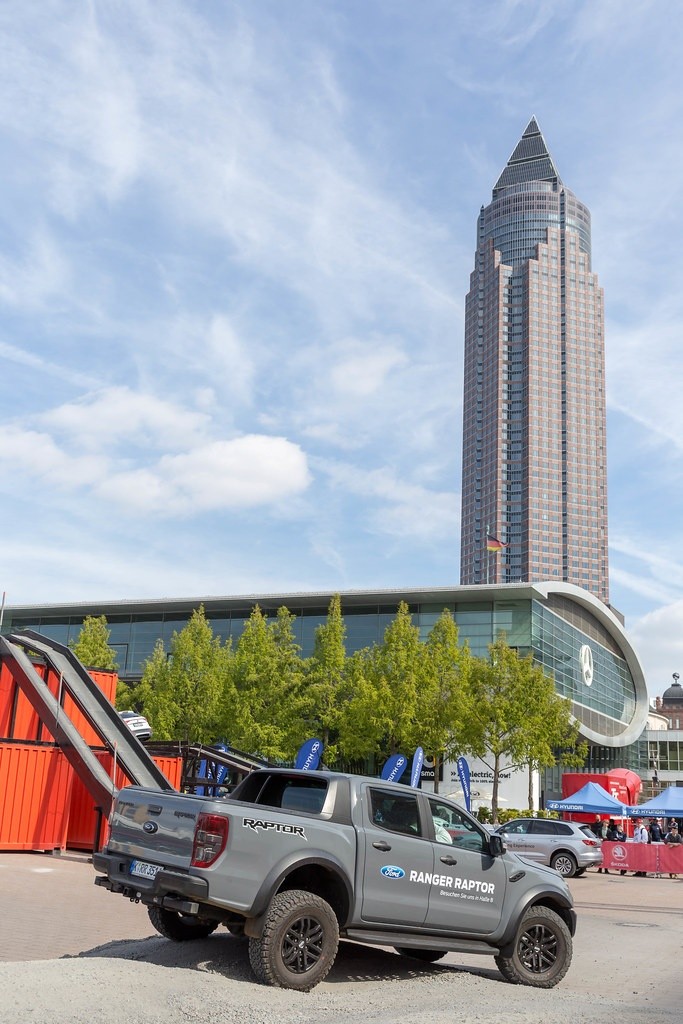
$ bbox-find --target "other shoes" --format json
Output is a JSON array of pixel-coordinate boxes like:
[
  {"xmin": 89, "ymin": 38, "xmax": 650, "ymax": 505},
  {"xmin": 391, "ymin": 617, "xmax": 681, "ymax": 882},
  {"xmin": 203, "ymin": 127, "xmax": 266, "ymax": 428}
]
[
  {"xmin": 633, "ymin": 871, "xmax": 647, "ymax": 876},
  {"xmin": 596, "ymin": 869, "xmax": 602, "ymax": 873},
  {"xmin": 604, "ymin": 870, "xmax": 610, "ymax": 873}
]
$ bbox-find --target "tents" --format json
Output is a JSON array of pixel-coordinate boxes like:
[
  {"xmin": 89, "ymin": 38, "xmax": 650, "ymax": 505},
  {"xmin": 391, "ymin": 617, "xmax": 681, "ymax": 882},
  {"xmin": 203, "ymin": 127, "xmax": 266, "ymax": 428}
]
[
  {"xmin": 546, "ymin": 782, "xmax": 629, "ymax": 838},
  {"xmin": 626, "ymin": 786, "xmax": 683, "ymax": 843}
]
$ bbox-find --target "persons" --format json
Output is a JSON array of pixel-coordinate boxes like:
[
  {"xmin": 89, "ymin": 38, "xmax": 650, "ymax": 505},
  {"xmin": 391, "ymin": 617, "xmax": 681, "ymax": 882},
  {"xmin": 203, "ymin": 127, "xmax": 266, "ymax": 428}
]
[
  {"xmin": 592, "ymin": 815, "xmax": 602, "ymax": 828},
  {"xmin": 406, "ymin": 805, "xmax": 452, "ymax": 844},
  {"xmin": 522, "ymin": 824, "xmax": 528, "ymax": 832},
  {"xmin": 645, "ymin": 817, "xmax": 678, "ymax": 843},
  {"xmin": 597, "ymin": 819, "xmax": 612, "ymax": 873},
  {"xmin": 633, "ymin": 819, "xmax": 649, "ymax": 877},
  {"xmin": 664, "ymin": 827, "xmax": 683, "ymax": 878},
  {"xmin": 611, "ymin": 825, "xmax": 627, "ymax": 876}
]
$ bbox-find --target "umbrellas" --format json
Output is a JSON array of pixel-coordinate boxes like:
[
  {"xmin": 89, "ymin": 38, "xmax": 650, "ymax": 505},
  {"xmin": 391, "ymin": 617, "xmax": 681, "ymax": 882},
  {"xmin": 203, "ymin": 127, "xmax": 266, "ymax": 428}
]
[{"xmin": 445, "ymin": 786, "xmax": 508, "ymax": 813}]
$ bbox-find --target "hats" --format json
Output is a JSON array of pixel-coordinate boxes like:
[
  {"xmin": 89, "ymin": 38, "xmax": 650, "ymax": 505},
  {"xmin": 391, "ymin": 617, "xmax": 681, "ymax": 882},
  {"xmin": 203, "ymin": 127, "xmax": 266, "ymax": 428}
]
[{"xmin": 636, "ymin": 818, "xmax": 643, "ymax": 824}]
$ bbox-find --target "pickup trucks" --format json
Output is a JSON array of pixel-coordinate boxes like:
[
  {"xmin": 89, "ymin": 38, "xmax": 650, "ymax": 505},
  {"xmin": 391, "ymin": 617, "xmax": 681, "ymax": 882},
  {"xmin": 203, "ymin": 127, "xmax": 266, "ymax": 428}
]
[{"xmin": 92, "ymin": 768, "xmax": 579, "ymax": 995}]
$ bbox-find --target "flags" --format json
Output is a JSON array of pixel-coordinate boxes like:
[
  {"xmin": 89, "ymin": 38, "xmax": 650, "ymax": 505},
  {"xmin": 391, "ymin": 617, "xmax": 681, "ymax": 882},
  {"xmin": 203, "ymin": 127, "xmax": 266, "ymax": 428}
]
[{"xmin": 487, "ymin": 534, "xmax": 509, "ymax": 552}]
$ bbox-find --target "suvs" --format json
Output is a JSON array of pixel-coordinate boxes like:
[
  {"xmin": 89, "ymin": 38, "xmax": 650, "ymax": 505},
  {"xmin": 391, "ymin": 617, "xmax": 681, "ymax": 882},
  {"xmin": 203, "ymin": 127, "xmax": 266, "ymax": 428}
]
[
  {"xmin": 118, "ymin": 710, "xmax": 152, "ymax": 742},
  {"xmin": 453, "ymin": 817, "xmax": 605, "ymax": 878}
]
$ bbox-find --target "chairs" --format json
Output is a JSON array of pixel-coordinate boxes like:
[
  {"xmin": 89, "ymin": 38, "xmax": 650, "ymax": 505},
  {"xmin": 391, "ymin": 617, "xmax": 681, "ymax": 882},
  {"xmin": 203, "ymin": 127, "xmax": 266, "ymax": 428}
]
[{"xmin": 385, "ymin": 799, "xmax": 416, "ymax": 835}]
